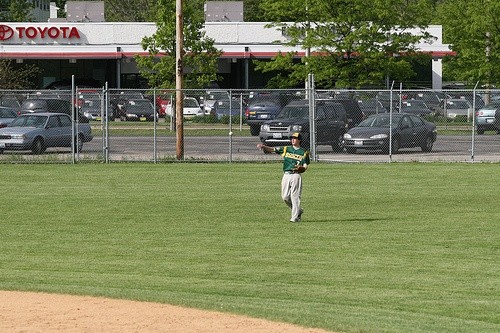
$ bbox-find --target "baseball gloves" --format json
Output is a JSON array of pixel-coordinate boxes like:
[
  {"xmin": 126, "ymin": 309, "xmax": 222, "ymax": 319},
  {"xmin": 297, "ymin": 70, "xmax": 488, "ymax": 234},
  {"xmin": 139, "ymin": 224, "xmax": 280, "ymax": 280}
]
[{"xmin": 290, "ymin": 165, "xmax": 305, "ymax": 173}]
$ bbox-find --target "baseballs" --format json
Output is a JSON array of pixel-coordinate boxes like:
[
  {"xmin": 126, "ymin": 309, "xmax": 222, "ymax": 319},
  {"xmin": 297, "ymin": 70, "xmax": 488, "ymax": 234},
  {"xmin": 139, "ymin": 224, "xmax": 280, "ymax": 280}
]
[{"xmin": 228, "ymin": 131, "xmax": 233, "ymax": 135}]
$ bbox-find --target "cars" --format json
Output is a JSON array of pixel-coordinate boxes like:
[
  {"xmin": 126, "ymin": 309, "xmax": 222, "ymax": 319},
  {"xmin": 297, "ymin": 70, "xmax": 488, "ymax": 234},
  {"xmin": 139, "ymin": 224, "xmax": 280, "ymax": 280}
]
[
  {"xmin": 354, "ymin": 97, "xmax": 388, "ymax": 120},
  {"xmin": 21, "ymin": 97, "xmax": 90, "ymax": 124},
  {"xmin": 151, "ymin": 92, "xmax": 185, "ymax": 117},
  {"xmin": 209, "ymin": 100, "xmax": 244, "ymax": 125},
  {"xmin": 164, "ymin": 97, "xmax": 205, "ymax": 122},
  {"xmin": 476, "ymin": 102, "xmax": 500, "ymax": 135},
  {"xmin": 393, "ymin": 100, "xmax": 436, "ymax": 121},
  {"xmin": 286, "ymin": 86, "xmax": 500, "ymax": 119},
  {"xmin": 432, "ymin": 99, "xmax": 475, "ymax": 122},
  {"xmin": 344, "ymin": 111, "xmax": 438, "ymax": 153},
  {"xmin": 78, "ymin": 99, "xmax": 116, "ymax": 122},
  {"xmin": 1, "ymin": 113, "xmax": 93, "ymax": 155},
  {"xmin": 199, "ymin": 89, "xmax": 231, "ymax": 116},
  {"xmin": 110, "ymin": 91, "xmax": 145, "ymax": 115},
  {"xmin": 120, "ymin": 99, "xmax": 160, "ymax": 123},
  {"xmin": 37, "ymin": 78, "xmax": 102, "ymax": 108},
  {"xmin": 0, "ymin": 106, "xmax": 18, "ymax": 128},
  {"xmin": 319, "ymin": 99, "xmax": 364, "ymax": 128}
]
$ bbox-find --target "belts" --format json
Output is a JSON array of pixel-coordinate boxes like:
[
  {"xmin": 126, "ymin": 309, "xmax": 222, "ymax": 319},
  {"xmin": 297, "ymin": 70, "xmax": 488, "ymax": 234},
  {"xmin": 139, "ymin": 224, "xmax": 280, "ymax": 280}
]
[{"xmin": 284, "ymin": 171, "xmax": 297, "ymax": 174}]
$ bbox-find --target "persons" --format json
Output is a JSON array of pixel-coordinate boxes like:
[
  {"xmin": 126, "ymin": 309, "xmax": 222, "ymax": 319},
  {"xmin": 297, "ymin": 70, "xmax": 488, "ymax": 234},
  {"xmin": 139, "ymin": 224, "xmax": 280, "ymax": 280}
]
[{"xmin": 257, "ymin": 133, "xmax": 310, "ymax": 223}]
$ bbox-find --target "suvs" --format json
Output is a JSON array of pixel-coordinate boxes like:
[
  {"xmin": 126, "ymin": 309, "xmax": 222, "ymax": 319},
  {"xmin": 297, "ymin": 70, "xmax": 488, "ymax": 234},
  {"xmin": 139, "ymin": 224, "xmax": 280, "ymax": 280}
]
[
  {"xmin": 244, "ymin": 91, "xmax": 300, "ymax": 137},
  {"xmin": 258, "ymin": 98, "xmax": 351, "ymax": 155}
]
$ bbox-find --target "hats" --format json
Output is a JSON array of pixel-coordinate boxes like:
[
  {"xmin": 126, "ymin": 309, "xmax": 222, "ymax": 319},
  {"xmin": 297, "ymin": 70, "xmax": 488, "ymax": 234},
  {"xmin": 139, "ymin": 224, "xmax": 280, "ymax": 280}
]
[{"xmin": 290, "ymin": 133, "xmax": 302, "ymax": 140}]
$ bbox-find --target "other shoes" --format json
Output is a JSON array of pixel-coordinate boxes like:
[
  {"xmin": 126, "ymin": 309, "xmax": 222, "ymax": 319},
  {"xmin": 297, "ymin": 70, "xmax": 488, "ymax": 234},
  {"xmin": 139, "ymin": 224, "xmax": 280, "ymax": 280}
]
[
  {"xmin": 290, "ymin": 218, "xmax": 298, "ymax": 222},
  {"xmin": 298, "ymin": 209, "xmax": 303, "ymax": 221}
]
